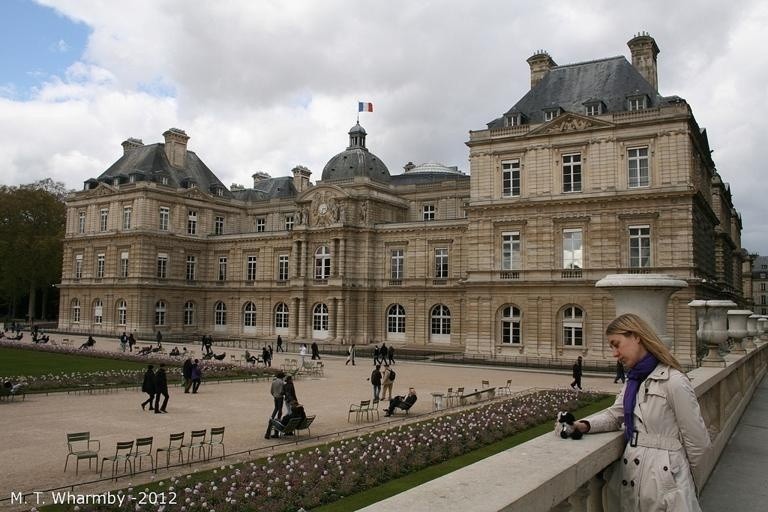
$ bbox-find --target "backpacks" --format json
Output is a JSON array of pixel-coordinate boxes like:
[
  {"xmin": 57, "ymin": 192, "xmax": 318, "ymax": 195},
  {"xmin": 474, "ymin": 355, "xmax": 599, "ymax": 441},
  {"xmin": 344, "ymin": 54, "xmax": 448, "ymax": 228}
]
[{"xmin": 386, "ymin": 368, "xmax": 395, "ymax": 381}]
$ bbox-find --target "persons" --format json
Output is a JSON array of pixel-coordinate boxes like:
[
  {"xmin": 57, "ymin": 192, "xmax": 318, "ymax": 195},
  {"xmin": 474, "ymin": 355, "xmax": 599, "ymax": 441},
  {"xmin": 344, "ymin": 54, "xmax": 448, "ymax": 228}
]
[
  {"xmin": 614, "ymin": 360, "xmax": 625, "ymax": 384},
  {"xmin": 572, "ymin": 312, "xmax": 713, "ymax": 511},
  {"xmin": 570, "ymin": 355, "xmax": 582, "ymax": 389},
  {"xmin": 0, "ymin": 316, "xmax": 418, "ymax": 440}
]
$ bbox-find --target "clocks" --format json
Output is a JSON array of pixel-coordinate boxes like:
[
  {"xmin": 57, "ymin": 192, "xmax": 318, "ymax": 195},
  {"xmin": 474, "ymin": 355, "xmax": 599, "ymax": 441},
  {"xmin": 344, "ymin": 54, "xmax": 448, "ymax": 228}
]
[{"xmin": 317, "ymin": 203, "xmax": 329, "ymax": 215}]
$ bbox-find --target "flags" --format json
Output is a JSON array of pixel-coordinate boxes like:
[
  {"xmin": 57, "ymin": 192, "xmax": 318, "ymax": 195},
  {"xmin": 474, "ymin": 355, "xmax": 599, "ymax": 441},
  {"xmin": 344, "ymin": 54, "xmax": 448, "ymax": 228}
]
[{"xmin": 358, "ymin": 101, "xmax": 373, "ymax": 114}]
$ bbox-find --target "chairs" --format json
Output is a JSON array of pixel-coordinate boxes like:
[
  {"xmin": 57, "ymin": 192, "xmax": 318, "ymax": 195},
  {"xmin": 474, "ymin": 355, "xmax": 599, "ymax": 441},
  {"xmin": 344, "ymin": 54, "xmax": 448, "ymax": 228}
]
[
  {"xmin": 0, "ymin": 325, "xmax": 327, "ymax": 403},
  {"xmin": 428, "ymin": 377, "xmax": 515, "ymax": 415},
  {"xmin": 346, "ymin": 400, "xmax": 369, "ymax": 426},
  {"xmin": 359, "ymin": 397, "xmax": 382, "ymax": 423},
  {"xmin": 63, "ymin": 424, "xmax": 228, "ymax": 483},
  {"xmin": 297, "ymin": 413, "xmax": 317, "ymax": 439},
  {"xmin": 270, "ymin": 415, "xmax": 299, "ymax": 443}
]
